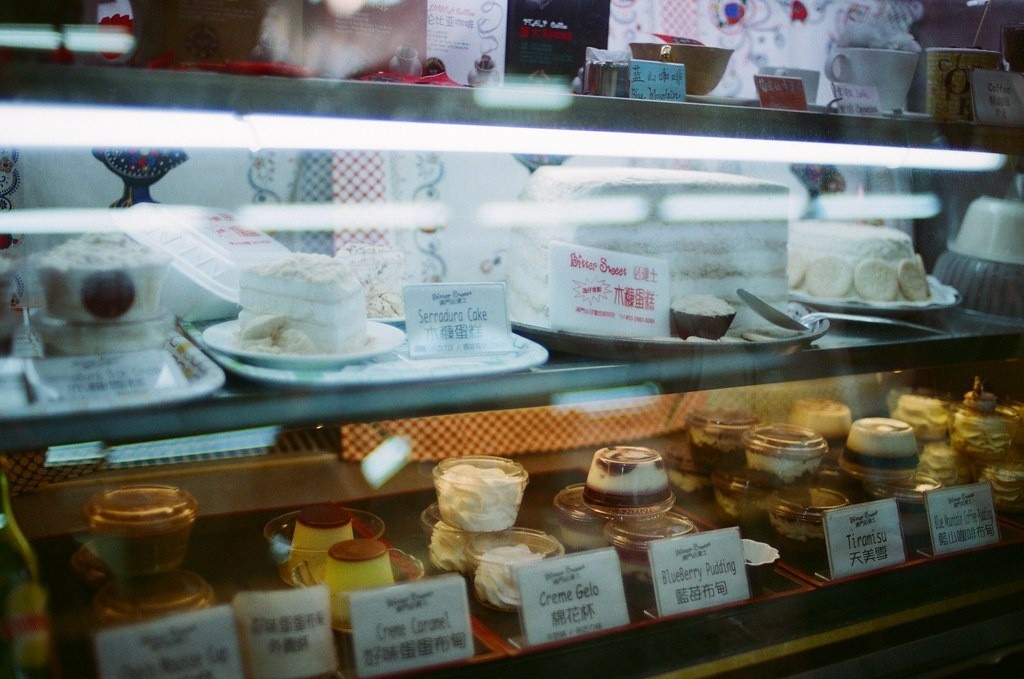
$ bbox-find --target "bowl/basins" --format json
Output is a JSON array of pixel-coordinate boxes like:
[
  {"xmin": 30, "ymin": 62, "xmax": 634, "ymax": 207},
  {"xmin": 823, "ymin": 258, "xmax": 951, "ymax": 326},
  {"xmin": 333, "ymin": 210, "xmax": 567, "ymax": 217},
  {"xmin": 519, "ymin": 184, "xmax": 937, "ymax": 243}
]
[
  {"xmin": 582, "ymin": 444, "xmax": 675, "ymax": 518},
  {"xmin": 603, "ymin": 511, "xmax": 698, "ymax": 594},
  {"xmin": 660, "ymin": 385, "xmax": 1024, "ymax": 571},
  {"xmin": 420, "ymin": 500, "xmax": 476, "ymax": 579},
  {"xmin": 432, "ymin": 455, "xmax": 529, "ymax": 534},
  {"xmin": 629, "ymin": 42, "xmax": 735, "ymax": 95},
  {"xmin": 552, "ymin": 481, "xmax": 611, "ymax": 551},
  {"xmin": 263, "ymin": 508, "xmax": 385, "ymax": 588},
  {"xmin": 948, "ymin": 194, "xmax": 1024, "ymax": 265},
  {"xmin": 91, "ymin": 571, "xmax": 216, "ymax": 627},
  {"xmin": 464, "ymin": 527, "xmax": 565, "ymax": 613},
  {"xmin": 290, "ymin": 547, "xmax": 425, "ymax": 634},
  {"xmin": 83, "ymin": 482, "xmax": 199, "ymax": 598}
]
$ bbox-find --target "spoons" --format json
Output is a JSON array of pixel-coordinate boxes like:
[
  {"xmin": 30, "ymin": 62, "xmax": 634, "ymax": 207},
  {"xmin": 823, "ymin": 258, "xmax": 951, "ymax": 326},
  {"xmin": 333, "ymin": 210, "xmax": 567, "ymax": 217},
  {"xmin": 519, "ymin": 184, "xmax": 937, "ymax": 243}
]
[{"xmin": 735, "ymin": 287, "xmax": 949, "ymax": 337}]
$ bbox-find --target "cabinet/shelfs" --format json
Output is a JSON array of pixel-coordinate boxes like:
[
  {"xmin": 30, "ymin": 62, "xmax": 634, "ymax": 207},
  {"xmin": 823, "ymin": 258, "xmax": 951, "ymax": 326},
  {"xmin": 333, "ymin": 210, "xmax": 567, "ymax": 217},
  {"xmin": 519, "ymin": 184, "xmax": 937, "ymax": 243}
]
[{"xmin": 0, "ymin": 57, "xmax": 1024, "ymax": 679}]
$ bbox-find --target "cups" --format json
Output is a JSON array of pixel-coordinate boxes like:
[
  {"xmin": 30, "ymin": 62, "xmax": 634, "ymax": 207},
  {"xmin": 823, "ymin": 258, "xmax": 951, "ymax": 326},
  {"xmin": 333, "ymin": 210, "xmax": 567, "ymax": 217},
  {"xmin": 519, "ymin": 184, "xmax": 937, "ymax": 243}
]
[
  {"xmin": 824, "ymin": 48, "xmax": 919, "ymax": 111},
  {"xmin": 396, "ymin": 45, "xmax": 418, "ymax": 74},
  {"xmin": 925, "ymin": 47, "xmax": 1002, "ymax": 121},
  {"xmin": 999, "ymin": 22, "xmax": 1024, "ymax": 73}
]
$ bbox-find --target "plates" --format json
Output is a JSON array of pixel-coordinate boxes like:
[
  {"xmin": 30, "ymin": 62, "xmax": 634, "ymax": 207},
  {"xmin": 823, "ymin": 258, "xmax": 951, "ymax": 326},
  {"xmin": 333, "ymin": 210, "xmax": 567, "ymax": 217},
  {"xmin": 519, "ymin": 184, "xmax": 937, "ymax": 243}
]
[
  {"xmin": 789, "ymin": 277, "xmax": 963, "ymax": 323},
  {"xmin": 203, "ymin": 319, "xmax": 408, "ymax": 371},
  {"xmin": 685, "ymin": 94, "xmax": 755, "ymax": 105}
]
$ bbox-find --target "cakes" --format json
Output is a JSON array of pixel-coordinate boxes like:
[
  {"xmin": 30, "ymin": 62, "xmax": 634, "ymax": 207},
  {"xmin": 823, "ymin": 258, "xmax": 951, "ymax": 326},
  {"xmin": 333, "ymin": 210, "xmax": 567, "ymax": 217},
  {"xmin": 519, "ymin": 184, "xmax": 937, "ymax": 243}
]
[{"xmin": 498, "ymin": 165, "xmax": 1024, "ymax": 338}]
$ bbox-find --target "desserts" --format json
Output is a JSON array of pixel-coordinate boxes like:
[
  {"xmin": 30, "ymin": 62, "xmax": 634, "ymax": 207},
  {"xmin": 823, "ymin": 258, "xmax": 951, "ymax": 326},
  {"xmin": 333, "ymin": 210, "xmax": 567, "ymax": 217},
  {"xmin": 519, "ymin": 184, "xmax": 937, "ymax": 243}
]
[
  {"xmin": 33, "ymin": 227, "xmax": 408, "ymax": 356},
  {"xmin": 72, "ymin": 375, "xmax": 1024, "ymax": 624}
]
